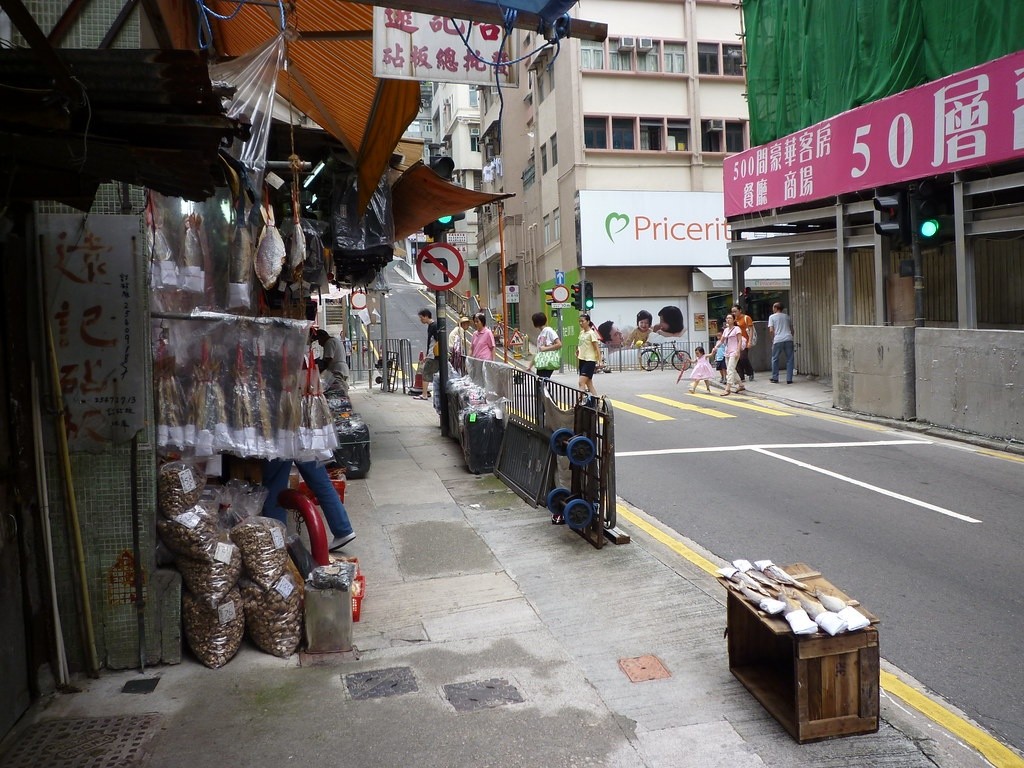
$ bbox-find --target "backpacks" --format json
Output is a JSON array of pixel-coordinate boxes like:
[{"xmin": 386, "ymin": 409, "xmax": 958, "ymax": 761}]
[{"xmin": 726, "ymin": 327, "xmax": 747, "ymax": 351}]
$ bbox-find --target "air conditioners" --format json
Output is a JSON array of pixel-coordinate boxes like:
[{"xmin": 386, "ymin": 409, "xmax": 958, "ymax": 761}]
[
  {"xmin": 484, "ymin": 136, "xmax": 493, "ymax": 145},
  {"xmin": 487, "ymin": 145, "xmax": 495, "ymax": 160},
  {"xmin": 636, "ymin": 37, "xmax": 653, "ymax": 52},
  {"xmin": 618, "ymin": 36, "xmax": 636, "ymax": 52},
  {"xmin": 705, "ymin": 120, "xmax": 723, "ymax": 133},
  {"xmin": 443, "ymin": 97, "xmax": 451, "ymax": 106},
  {"xmin": 470, "ymin": 128, "xmax": 479, "ymax": 136}
]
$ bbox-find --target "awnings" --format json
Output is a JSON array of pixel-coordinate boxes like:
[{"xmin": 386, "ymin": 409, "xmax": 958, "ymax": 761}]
[
  {"xmin": 198, "ymin": 0, "xmax": 428, "ymax": 225},
  {"xmin": 383, "ymin": 158, "xmax": 516, "ymax": 242}
]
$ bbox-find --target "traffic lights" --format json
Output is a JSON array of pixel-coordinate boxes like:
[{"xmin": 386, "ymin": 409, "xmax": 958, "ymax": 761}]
[
  {"xmin": 453, "ymin": 208, "xmax": 465, "ymax": 222},
  {"xmin": 916, "ymin": 178, "xmax": 945, "ymax": 245},
  {"xmin": 873, "ymin": 183, "xmax": 911, "ymax": 250},
  {"xmin": 584, "ymin": 282, "xmax": 595, "ymax": 309},
  {"xmin": 545, "ymin": 288, "xmax": 555, "ymax": 305},
  {"xmin": 430, "ymin": 155, "xmax": 455, "ymax": 230},
  {"xmin": 571, "ymin": 282, "xmax": 582, "ymax": 310},
  {"xmin": 552, "ymin": 310, "xmax": 558, "ymax": 316}
]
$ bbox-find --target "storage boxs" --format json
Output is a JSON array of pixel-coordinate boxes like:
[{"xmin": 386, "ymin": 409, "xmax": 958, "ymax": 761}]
[
  {"xmin": 727, "ymin": 590, "xmax": 881, "ymax": 745},
  {"xmin": 299, "ymin": 472, "xmax": 346, "ymax": 504},
  {"xmin": 301, "ymin": 558, "xmax": 365, "ymax": 622}
]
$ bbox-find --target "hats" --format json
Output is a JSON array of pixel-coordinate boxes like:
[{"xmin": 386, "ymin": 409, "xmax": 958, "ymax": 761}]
[{"xmin": 457, "ymin": 317, "xmax": 474, "ymax": 326}]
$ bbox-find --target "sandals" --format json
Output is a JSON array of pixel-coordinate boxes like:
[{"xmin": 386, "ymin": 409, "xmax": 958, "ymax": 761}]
[
  {"xmin": 720, "ymin": 389, "xmax": 731, "ymax": 396},
  {"xmin": 735, "ymin": 385, "xmax": 745, "ymax": 393}
]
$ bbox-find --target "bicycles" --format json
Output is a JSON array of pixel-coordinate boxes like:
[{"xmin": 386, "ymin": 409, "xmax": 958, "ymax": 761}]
[{"xmin": 639, "ymin": 340, "xmax": 691, "ymax": 371}]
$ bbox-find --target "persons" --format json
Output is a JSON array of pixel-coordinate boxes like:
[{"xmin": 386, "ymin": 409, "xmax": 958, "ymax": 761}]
[
  {"xmin": 468, "ymin": 313, "xmax": 495, "ymax": 361},
  {"xmin": 653, "ymin": 306, "xmax": 685, "ymax": 336},
  {"xmin": 231, "ymin": 325, "xmax": 357, "ymax": 551},
  {"xmin": 413, "ymin": 309, "xmax": 437, "ymax": 400},
  {"xmin": 340, "ymin": 331, "xmax": 353, "ymax": 369},
  {"xmin": 315, "ymin": 329, "xmax": 350, "ymax": 380},
  {"xmin": 714, "ymin": 314, "xmax": 745, "ymax": 396},
  {"xmin": 449, "ymin": 317, "xmax": 472, "ymax": 355},
  {"xmin": 716, "ymin": 328, "xmax": 727, "ymax": 383},
  {"xmin": 623, "ymin": 310, "xmax": 653, "ymax": 349},
  {"xmin": 686, "ymin": 347, "xmax": 715, "ymax": 394},
  {"xmin": 768, "ymin": 302, "xmax": 795, "ymax": 383},
  {"xmin": 575, "ymin": 314, "xmax": 601, "ymax": 404},
  {"xmin": 732, "ymin": 305, "xmax": 754, "ymax": 381},
  {"xmin": 597, "ymin": 320, "xmax": 632, "ymax": 352},
  {"xmin": 525, "ymin": 312, "xmax": 562, "ymax": 418}
]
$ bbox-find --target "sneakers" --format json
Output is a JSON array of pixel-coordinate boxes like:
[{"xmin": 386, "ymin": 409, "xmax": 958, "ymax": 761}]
[{"xmin": 328, "ymin": 530, "xmax": 356, "ymax": 552}]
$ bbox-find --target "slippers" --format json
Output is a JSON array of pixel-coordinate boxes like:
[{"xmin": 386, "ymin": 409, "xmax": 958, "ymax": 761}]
[{"xmin": 413, "ymin": 395, "xmax": 428, "ymax": 400}]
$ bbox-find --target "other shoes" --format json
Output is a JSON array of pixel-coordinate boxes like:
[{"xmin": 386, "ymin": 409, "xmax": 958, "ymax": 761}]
[
  {"xmin": 742, "ymin": 380, "xmax": 746, "ymax": 382},
  {"xmin": 770, "ymin": 379, "xmax": 778, "ymax": 383},
  {"xmin": 787, "ymin": 381, "xmax": 793, "ymax": 384},
  {"xmin": 749, "ymin": 373, "xmax": 754, "ymax": 381},
  {"xmin": 720, "ymin": 378, "xmax": 724, "ymax": 383},
  {"xmin": 723, "ymin": 379, "xmax": 727, "ymax": 384}
]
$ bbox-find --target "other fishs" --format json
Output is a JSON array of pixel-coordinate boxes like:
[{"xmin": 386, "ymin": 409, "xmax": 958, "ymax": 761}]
[
  {"xmin": 754, "ymin": 559, "xmax": 806, "ymax": 590},
  {"xmin": 732, "ymin": 559, "xmax": 781, "ymax": 592},
  {"xmin": 717, "ymin": 567, "xmax": 786, "ymax": 616},
  {"xmin": 778, "ymin": 584, "xmax": 818, "ymax": 634},
  {"xmin": 790, "ymin": 589, "xmax": 848, "ymax": 636},
  {"xmin": 806, "ymin": 586, "xmax": 870, "ymax": 631}
]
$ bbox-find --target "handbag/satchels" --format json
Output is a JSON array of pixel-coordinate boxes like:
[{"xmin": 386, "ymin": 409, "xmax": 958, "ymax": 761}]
[
  {"xmin": 745, "ymin": 315, "xmax": 758, "ymax": 346},
  {"xmin": 533, "ymin": 331, "xmax": 561, "ymax": 370}
]
